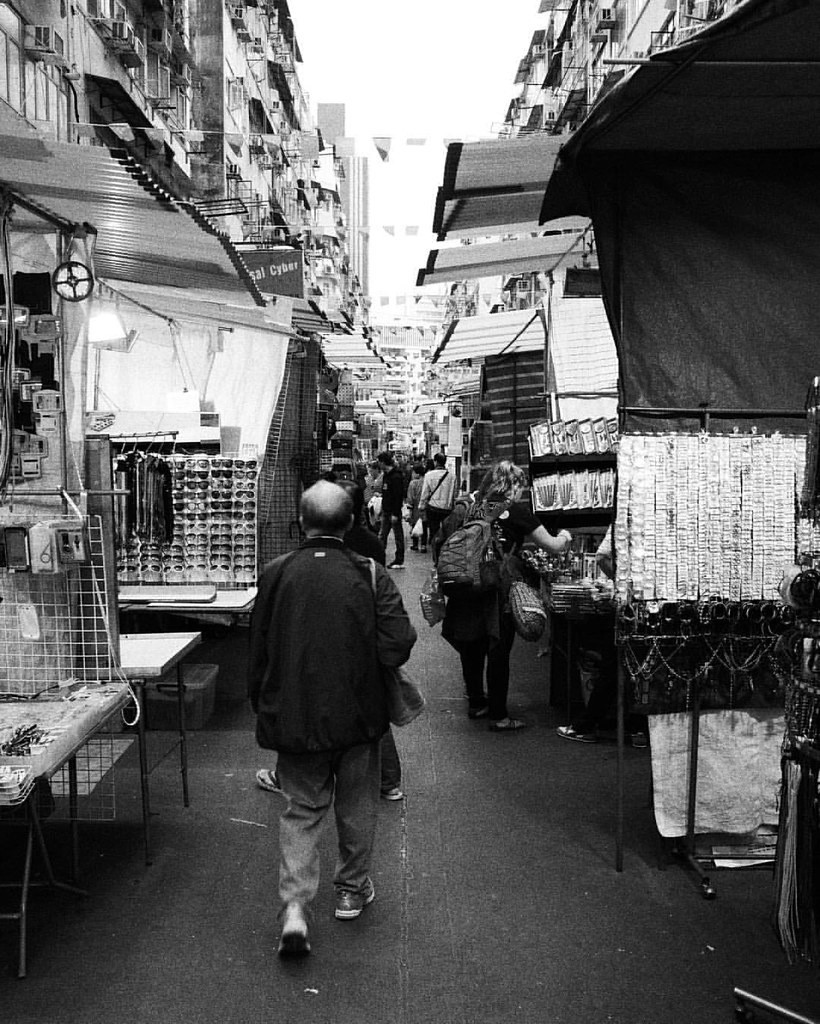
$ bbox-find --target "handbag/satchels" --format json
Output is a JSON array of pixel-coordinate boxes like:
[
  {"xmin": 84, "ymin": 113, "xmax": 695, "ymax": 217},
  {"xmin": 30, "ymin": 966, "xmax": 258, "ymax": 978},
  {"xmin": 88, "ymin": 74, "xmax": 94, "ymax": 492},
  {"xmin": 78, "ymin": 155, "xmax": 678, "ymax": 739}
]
[
  {"xmin": 513, "ymin": 580, "xmax": 547, "ymax": 643},
  {"xmin": 420, "ymin": 494, "xmax": 431, "ymax": 512},
  {"xmin": 410, "ymin": 516, "xmax": 423, "ymax": 538},
  {"xmin": 388, "ymin": 664, "xmax": 426, "ymax": 725},
  {"xmin": 366, "ymin": 494, "xmax": 383, "ymax": 516},
  {"xmin": 418, "ymin": 568, "xmax": 446, "ymax": 628}
]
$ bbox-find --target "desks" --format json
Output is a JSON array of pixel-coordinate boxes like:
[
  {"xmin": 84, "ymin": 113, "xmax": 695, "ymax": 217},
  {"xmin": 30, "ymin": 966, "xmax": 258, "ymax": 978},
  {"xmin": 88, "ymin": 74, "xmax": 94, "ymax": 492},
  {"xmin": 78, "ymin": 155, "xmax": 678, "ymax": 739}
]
[
  {"xmin": 69, "ymin": 632, "xmax": 203, "ymax": 823},
  {"xmin": 0, "ymin": 683, "xmax": 134, "ymax": 987},
  {"xmin": 119, "ymin": 591, "xmax": 261, "ymax": 698}
]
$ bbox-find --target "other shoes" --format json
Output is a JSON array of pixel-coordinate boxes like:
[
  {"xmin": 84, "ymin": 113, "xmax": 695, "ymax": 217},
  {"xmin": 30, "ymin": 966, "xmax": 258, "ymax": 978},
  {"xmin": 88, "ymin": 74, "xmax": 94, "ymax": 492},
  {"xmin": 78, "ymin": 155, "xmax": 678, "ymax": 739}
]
[
  {"xmin": 467, "ymin": 705, "xmax": 490, "ymax": 720},
  {"xmin": 421, "ymin": 548, "xmax": 427, "ymax": 553},
  {"xmin": 386, "ymin": 563, "xmax": 405, "ymax": 569},
  {"xmin": 410, "ymin": 546, "xmax": 418, "ymax": 550},
  {"xmin": 630, "ymin": 730, "xmax": 648, "ymax": 749},
  {"xmin": 556, "ymin": 725, "xmax": 596, "ymax": 745},
  {"xmin": 490, "ymin": 716, "xmax": 527, "ymax": 730}
]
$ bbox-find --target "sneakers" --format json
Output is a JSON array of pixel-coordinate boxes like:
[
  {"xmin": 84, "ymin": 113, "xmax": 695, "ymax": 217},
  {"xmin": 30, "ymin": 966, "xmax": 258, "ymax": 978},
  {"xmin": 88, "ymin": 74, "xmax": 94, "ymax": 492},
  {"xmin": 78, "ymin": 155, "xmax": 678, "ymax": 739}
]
[
  {"xmin": 334, "ymin": 875, "xmax": 374, "ymax": 918},
  {"xmin": 275, "ymin": 905, "xmax": 312, "ymax": 959}
]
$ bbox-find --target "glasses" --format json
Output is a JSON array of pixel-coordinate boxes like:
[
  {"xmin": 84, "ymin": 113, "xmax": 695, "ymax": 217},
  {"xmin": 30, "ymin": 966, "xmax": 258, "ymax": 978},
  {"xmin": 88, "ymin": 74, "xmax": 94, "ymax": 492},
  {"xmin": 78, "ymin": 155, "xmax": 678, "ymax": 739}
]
[{"xmin": 111, "ymin": 458, "xmax": 257, "ymax": 573}]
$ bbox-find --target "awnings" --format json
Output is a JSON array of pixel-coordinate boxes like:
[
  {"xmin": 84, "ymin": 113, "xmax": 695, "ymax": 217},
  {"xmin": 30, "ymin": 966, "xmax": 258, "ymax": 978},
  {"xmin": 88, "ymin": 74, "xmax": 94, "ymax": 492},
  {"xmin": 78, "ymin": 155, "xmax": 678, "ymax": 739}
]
[
  {"xmin": 414, "ymin": 134, "xmax": 596, "ymax": 286},
  {"xmin": 431, "ymin": 308, "xmax": 545, "ymax": 364},
  {"xmin": 0, "ymin": 133, "xmax": 269, "ymax": 308}
]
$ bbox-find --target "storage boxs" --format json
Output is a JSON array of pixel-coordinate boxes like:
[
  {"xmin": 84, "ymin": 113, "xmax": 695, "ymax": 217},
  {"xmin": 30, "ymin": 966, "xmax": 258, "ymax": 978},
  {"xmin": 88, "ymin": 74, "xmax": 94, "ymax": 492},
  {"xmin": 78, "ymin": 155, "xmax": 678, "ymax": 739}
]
[{"xmin": 146, "ymin": 663, "xmax": 219, "ymax": 731}]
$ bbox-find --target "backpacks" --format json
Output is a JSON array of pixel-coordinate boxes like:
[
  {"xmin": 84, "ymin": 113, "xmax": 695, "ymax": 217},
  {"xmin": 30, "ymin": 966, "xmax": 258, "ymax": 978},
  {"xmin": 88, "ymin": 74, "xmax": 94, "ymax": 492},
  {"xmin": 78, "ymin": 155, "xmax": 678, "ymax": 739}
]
[{"xmin": 436, "ymin": 494, "xmax": 514, "ymax": 598}]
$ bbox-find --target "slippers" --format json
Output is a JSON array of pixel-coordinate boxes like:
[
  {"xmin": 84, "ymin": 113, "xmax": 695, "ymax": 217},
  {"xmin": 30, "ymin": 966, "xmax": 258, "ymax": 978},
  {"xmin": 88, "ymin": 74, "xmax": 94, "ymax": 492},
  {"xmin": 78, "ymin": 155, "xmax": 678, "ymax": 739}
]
[
  {"xmin": 257, "ymin": 768, "xmax": 282, "ymax": 793},
  {"xmin": 383, "ymin": 787, "xmax": 404, "ymax": 802}
]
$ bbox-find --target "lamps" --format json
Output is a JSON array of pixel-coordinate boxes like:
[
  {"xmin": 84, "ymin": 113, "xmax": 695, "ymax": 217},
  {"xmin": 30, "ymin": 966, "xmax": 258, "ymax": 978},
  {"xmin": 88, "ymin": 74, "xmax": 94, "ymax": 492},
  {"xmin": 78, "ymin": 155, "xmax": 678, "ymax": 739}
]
[
  {"xmin": 171, "ymin": 130, "xmax": 204, "ymax": 142},
  {"xmin": 450, "ymin": 405, "xmax": 478, "ymax": 416}
]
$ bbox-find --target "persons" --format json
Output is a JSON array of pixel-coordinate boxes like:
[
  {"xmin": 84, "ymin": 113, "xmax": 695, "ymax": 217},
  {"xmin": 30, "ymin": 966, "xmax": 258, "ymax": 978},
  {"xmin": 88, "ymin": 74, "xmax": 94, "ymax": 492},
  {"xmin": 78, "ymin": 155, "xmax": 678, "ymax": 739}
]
[
  {"xmin": 429, "ymin": 461, "xmax": 573, "ymax": 731},
  {"xmin": 406, "ymin": 453, "xmax": 455, "ymax": 553},
  {"xmin": 557, "ymin": 524, "xmax": 647, "ymax": 748},
  {"xmin": 364, "ymin": 452, "xmax": 406, "ymax": 569},
  {"xmin": 245, "ymin": 481, "xmax": 419, "ymax": 961}
]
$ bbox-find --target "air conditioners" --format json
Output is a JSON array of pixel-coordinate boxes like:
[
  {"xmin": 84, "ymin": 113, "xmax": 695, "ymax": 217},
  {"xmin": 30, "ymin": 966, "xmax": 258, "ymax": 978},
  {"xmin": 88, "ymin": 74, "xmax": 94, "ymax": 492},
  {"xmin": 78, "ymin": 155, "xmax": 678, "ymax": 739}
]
[
  {"xmin": 515, "ymin": 279, "xmax": 531, "ymax": 291},
  {"xmin": 25, "ymin": 5, "xmax": 311, "ymax": 242},
  {"xmin": 533, "ymin": 7, "xmax": 643, "ymax": 124}
]
